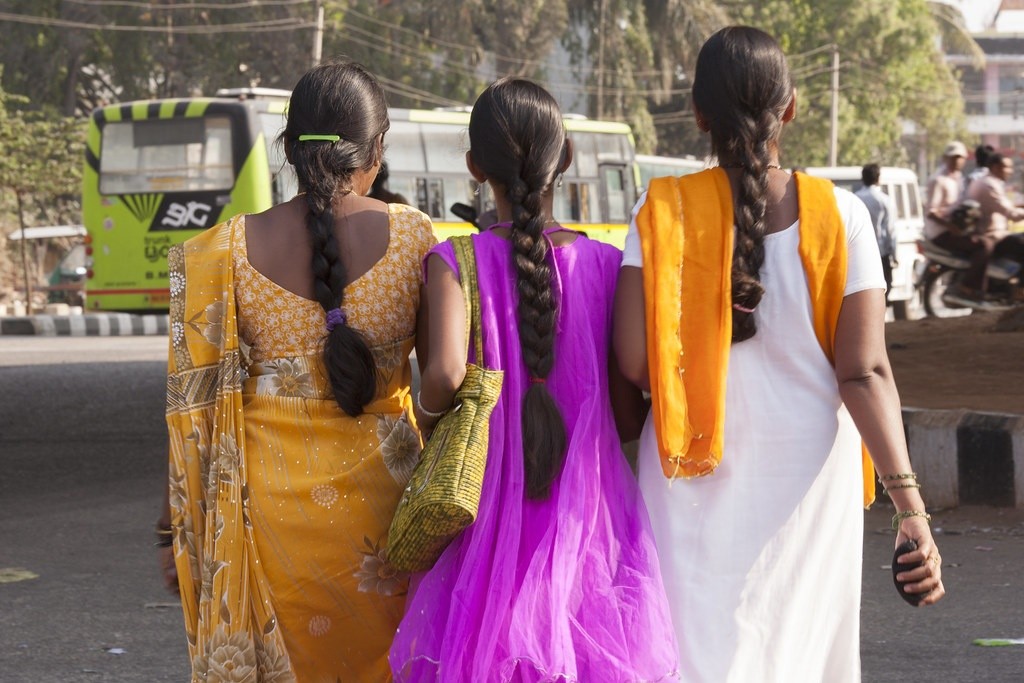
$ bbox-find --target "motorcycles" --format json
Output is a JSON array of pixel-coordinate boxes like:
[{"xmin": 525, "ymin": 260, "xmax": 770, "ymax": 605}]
[{"xmin": 915, "ymin": 230, "xmax": 1024, "ymax": 321}]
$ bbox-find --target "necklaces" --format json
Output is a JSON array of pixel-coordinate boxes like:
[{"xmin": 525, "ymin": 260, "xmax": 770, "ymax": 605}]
[
  {"xmin": 766, "ymin": 162, "xmax": 782, "ymax": 170},
  {"xmin": 501, "ymin": 219, "xmax": 557, "ymax": 225},
  {"xmin": 292, "ymin": 189, "xmax": 357, "ymax": 199}
]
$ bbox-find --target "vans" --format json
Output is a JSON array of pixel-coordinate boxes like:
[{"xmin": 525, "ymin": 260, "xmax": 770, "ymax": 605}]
[{"xmin": 804, "ymin": 166, "xmax": 945, "ymax": 323}]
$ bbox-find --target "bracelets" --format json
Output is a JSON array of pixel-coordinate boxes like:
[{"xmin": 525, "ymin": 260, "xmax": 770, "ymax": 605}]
[
  {"xmin": 883, "ymin": 482, "xmax": 921, "ymax": 494},
  {"xmin": 877, "ymin": 472, "xmax": 917, "ymax": 484},
  {"xmin": 416, "ymin": 389, "xmax": 445, "ymax": 419},
  {"xmin": 890, "ymin": 510, "xmax": 932, "ymax": 531}
]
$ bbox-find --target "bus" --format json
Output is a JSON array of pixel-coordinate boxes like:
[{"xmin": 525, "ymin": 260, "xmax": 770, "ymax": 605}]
[{"xmin": 81, "ymin": 85, "xmax": 648, "ymax": 318}]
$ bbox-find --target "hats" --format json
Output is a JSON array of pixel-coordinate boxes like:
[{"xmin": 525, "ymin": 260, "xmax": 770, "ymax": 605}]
[{"xmin": 944, "ymin": 142, "xmax": 969, "ymax": 159}]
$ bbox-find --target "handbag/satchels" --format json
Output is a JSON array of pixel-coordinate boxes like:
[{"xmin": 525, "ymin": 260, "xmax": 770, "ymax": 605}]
[{"xmin": 385, "ymin": 233, "xmax": 506, "ymax": 573}]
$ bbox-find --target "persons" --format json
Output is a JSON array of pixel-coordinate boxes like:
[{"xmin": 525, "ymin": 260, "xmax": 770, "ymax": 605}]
[
  {"xmin": 168, "ymin": 65, "xmax": 447, "ymax": 682},
  {"xmin": 611, "ymin": 25, "xmax": 944, "ymax": 683},
  {"xmin": 853, "ymin": 142, "xmax": 1024, "ymax": 319},
  {"xmin": 413, "ymin": 73, "xmax": 649, "ymax": 683}
]
